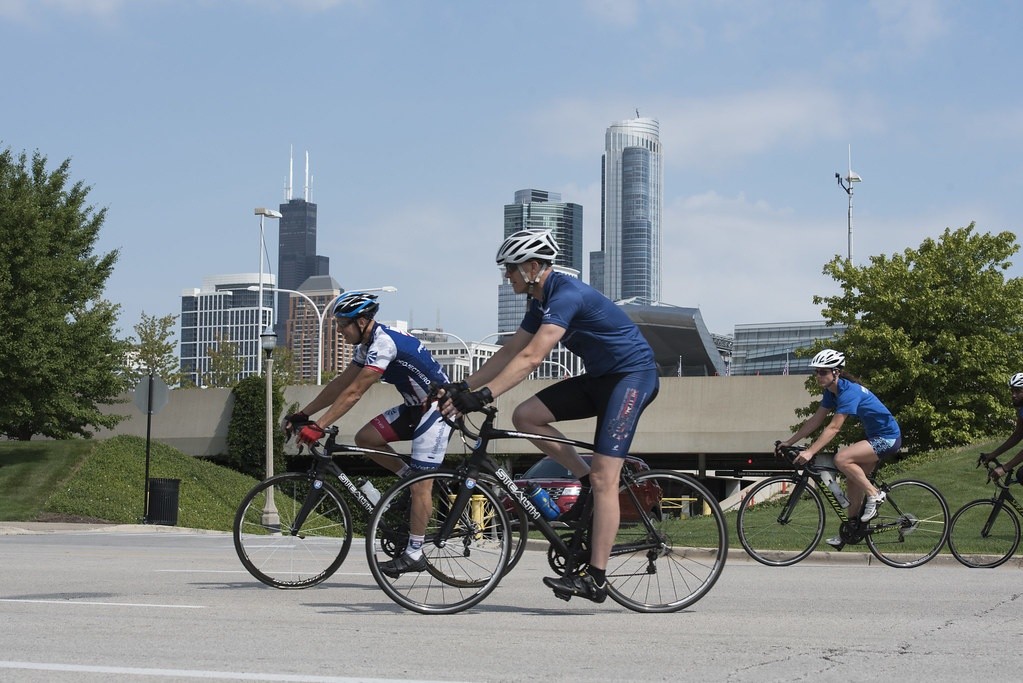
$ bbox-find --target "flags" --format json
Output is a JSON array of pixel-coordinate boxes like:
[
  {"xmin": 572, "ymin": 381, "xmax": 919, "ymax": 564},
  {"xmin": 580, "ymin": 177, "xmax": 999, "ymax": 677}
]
[{"xmin": 782, "ymin": 361, "xmax": 787, "ymax": 375}]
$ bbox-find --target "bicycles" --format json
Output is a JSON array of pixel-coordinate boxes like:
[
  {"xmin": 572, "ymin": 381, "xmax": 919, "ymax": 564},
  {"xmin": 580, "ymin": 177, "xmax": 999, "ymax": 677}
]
[
  {"xmin": 232, "ymin": 421, "xmax": 529, "ymax": 590},
  {"xmin": 737, "ymin": 439, "xmax": 951, "ymax": 569},
  {"xmin": 946, "ymin": 453, "xmax": 1023, "ymax": 568},
  {"xmin": 365, "ymin": 387, "xmax": 730, "ymax": 616}
]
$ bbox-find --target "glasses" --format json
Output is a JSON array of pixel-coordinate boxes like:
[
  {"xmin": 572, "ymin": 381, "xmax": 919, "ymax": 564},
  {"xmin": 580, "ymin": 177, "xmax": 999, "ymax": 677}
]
[
  {"xmin": 506, "ymin": 259, "xmax": 540, "ymax": 273},
  {"xmin": 337, "ymin": 314, "xmax": 364, "ymax": 329},
  {"xmin": 1011, "ymin": 389, "xmax": 1023, "ymax": 394},
  {"xmin": 814, "ymin": 369, "xmax": 838, "ymax": 376}
]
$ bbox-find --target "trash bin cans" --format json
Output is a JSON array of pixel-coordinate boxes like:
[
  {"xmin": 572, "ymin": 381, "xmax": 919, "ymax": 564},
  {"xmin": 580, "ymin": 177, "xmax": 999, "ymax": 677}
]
[{"xmin": 148, "ymin": 478, "xmax": 182, "ymax": 526}]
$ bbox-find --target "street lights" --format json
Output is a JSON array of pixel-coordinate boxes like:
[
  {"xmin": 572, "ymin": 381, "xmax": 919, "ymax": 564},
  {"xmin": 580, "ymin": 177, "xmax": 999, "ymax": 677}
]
[
  {"xmin": 411, "ymin": 330, "xmax": 516, "ymax": 378},
  {"xmin": 245, "ymin": 285, "xmax": 397, "ymax": 384},
  {"xmin": 254, "ymin": 207, "xmax": 283, "ymax": 375},
  {"xmin": 260, "ymin": 325, "xmax": 283, "ymax": 537},
  {"xmin": 845, "ymin": 171, "xmax": 862, "ymax": 269}
]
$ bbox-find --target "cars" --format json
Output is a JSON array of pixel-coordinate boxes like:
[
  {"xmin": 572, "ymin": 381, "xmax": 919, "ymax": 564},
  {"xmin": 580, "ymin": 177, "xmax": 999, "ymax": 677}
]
[{"xmin": 497, "ymin": 452, "xmax": 663, "ymax": 534}]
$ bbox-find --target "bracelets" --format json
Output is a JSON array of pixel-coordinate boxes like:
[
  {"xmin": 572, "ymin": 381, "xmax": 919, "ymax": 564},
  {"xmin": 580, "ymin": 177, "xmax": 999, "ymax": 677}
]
[{"xmin": 1001, "ymin": 465, "xmax": 1006, "ymax": 473}]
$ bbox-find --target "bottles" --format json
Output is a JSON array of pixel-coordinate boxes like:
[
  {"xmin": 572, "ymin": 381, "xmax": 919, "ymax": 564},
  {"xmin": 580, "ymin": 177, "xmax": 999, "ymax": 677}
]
[
  {"xmin": 525, "ymin": 481, "xmax": 561, "ymax": 521},
  {"xmin": 355, "ymin": 478, "xmax": 390, "ymax": 513},
  {"xmin": 828, "ymin": 479, "xmax": 850, "ymax": 508}
]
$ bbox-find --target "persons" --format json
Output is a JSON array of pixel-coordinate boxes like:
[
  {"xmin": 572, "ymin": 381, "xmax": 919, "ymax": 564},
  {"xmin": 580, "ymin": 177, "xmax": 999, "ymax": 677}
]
[
  {"xmin": 423, "ymin": 227, "xmax": 660, "ymax": 603},
  {"xmin": 282, "ymin": 291, "xmax": 463, "ymax": 572},
  {"xmin": 977, "ymin": 373, "xmax": 1023, "ymax": 487},
  {"xmin": 774, "ymin": 349, "xmax": 902, "ymax": 546}
]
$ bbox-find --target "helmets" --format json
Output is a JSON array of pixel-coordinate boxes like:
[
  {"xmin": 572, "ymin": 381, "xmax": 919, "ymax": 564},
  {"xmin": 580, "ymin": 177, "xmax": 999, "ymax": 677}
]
[
  {"xmin": 497, "ymin": 229, "xmax": 560, "ymax": 265},
  {"xmin": 809, "ymin": 349, "xmax": 845, "ymax": 368},
  {"xmin": 1010, "ymin": 373, "xmax": 1023, "ymax": 388},
  {"xmin": 332, "ymin": 292, "xmax": 379, "ymax": 319}
]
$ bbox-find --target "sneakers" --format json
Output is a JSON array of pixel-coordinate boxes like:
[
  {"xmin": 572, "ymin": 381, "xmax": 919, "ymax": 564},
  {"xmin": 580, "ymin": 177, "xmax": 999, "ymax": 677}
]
[
  {"xmin": 394, "ymin": 480, "xmax": 412, "ymax": 504},
  {"xmin": 543, "ymin": 569, "xmax": 607, "ymax": 604},
  {"xmin": 378, "ymin": 553, "xmax": 428, "ymax": 574},
  {"xmin": 861, "ymin": 490, "xmax": 886, "ymax": 523},
  {"xmin": 559, "ymin": 486, "xmax": 590, "ymax": 522},
  {"xmin": 826, "ymin": 535, "xmax": 843, "ymax": 545}
]
